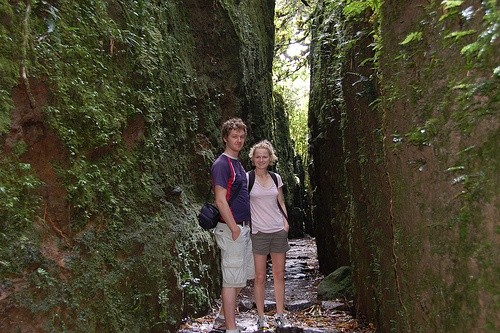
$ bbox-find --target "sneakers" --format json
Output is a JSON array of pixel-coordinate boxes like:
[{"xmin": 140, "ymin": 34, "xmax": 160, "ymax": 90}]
[
  {"xmin": 257, "ymin": 314, "xmax": 269, "ymax": 330},
  {"xmin": 212, "ymin": 316, "xmax": 247, "ymax": 331},
  {"xmin": 274, "ymin": 314, "xmax": 292, "ymax": 329}
]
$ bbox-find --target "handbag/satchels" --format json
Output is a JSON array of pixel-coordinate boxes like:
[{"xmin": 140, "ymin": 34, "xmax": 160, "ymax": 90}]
[{"xmin": 197, "ymin": 202, "xmax": 220, "ymax": 229}]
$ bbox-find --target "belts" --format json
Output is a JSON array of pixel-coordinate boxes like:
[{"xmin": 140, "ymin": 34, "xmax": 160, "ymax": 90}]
[{"xmin": 218, "ymin": 219, "xmax": 251, "ymax": 225}]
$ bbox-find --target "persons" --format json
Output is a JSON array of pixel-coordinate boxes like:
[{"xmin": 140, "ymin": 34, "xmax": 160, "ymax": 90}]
[
  {"xmin": 244, "ymin": 139, "xmax": 292, "ymax": 330},
  {"xmin": 211, "ymin": 117, "xmax": 256, "ymax": 333}
]
